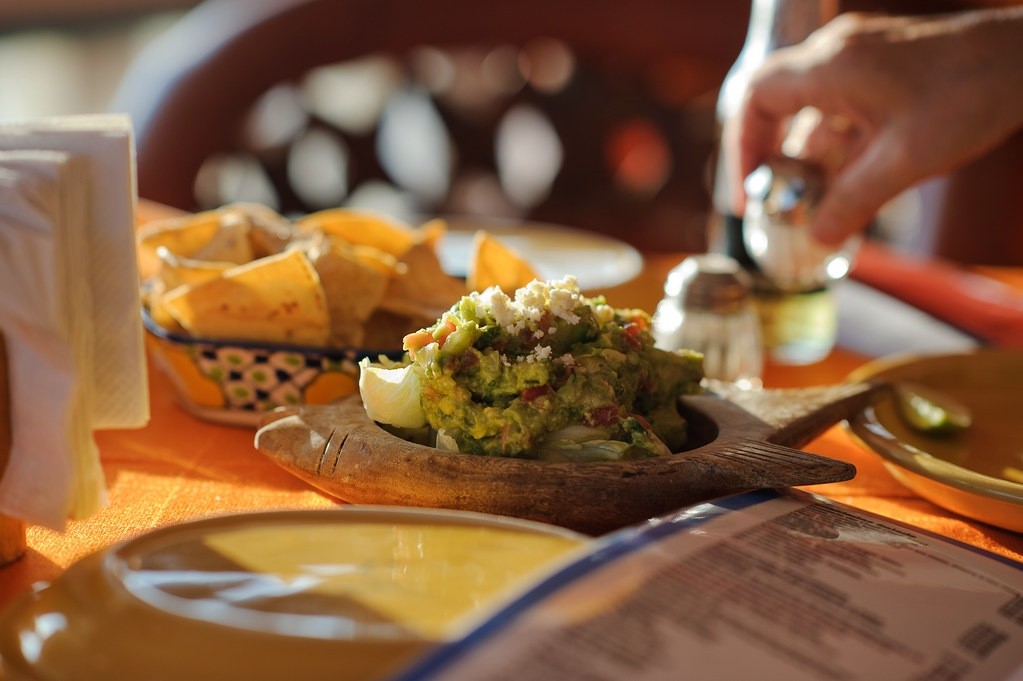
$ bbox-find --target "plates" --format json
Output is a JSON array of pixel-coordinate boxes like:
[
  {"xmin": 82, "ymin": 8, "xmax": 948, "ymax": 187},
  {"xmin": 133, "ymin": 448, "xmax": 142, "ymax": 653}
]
[
  {"xmin": 436, "ymin": 221, "xmax": 650, "ymax": 305},
  {"xmin": 858, "ymin": 347, "xmax": 1023, "ymax": 533},
  {"xmin": 241, "ymin": 380, "xmax": 878, "ymax": 519},
  {"xmin": 2, "ymin": 509, "xmax": 594, "ymax": 681}
]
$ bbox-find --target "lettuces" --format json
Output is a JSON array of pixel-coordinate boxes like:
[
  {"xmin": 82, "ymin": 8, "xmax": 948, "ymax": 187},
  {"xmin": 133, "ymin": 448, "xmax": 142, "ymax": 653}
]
[{"xmin": 357, "ymin": 295, "xmax": 706, "ymax": 461}]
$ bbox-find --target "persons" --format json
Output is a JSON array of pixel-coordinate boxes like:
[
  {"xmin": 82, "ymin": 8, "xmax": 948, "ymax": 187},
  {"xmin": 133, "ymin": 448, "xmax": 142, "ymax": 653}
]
[{"xmin": 716, "ymin": 0, "xmax": 1019, "ymax": 241}]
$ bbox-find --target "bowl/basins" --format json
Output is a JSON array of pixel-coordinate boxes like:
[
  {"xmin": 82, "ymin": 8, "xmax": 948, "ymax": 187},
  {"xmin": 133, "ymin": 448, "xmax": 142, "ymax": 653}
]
[{"xmin": 133, "ymin": 307, "xmax": 407, "ymax": 426}]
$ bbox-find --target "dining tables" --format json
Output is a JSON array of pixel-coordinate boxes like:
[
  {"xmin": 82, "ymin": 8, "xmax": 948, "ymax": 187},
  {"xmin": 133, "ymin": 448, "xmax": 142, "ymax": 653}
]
[{"xmin": 3, "ymin": 222, "xmax": 1021, "ymax": 680}]
